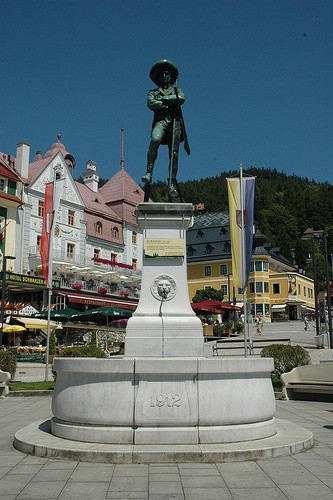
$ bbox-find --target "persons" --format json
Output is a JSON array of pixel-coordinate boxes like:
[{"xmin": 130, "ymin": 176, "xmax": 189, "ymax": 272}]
[
  {"xmin": 200, "ymin": 316, "xmax": 226, "ymax": 329},
  {"xmin": 257, "ymin": 316, "xmax": 262, "ymax": 328},
  {"xmin": 303, "ymin": 315, "xmax": 310, "ymax": 332},
  {"xmin": 256, "ymin": 318, "xmax": 264, "ymax": 335},
  {"xmin": 14, "ymin": 334, "xmax": 21, "ymax": 346},
  {"xmin": 118, "ymin": 342, "xmax": 125, "ymax": 355},
  {"xmin": 141, "ymin": 61, "xmax": 191, "ymax": 196},
  {"xmin": 229, "ymin": 312, "xmax": 233, "ymax": 320},
  {"xmin": 240, "ymin": 313, "xmax": 244, "ymax": 324}
]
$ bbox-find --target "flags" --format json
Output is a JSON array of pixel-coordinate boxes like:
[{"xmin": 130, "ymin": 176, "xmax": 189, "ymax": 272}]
[
  {"xmin": 226, "ymin": 178, "xmax": 255, "ymax": 290},
  {"xmin": 41, "ymin": 179, "xmax": 66, "ymax": 291}
]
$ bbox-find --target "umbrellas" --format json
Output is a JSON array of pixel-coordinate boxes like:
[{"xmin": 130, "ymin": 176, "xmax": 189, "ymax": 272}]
[
  {"xmin": 190, "ymin": 303, "xmax": 225, "ymax": 316},
  {"xmin": 2, "ymin": 325, "xmax": 26, "ymax": 347},
  {"xmin": 32, "ymin": 307, "xmax": 88, "ymax": 342},
  {"xmin": 197, "ymin": 300, "xmax": 243, "ymax": 312},
  {"xmin": 81, "ymin": 307, "xmax": 134, "ymax": 353},
  {"xmin": 113, "ymin": 320, "xmax": 128, "ymax": 324}
]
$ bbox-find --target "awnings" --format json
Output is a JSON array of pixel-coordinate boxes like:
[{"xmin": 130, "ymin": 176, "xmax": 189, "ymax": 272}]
[
  {"xmin": 14, "ymin": 317, "xmax": 59, "ymax": 331},
  {"xmin": 59, "ymin": 290, "xmax": 139, "ymax": 312}
]
